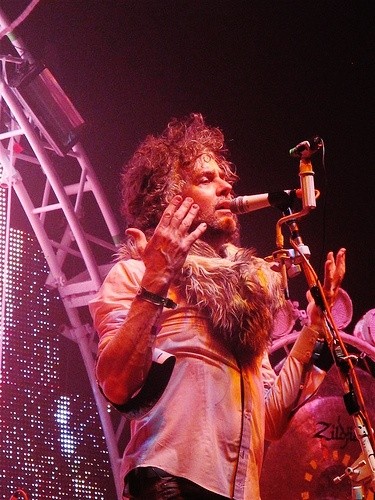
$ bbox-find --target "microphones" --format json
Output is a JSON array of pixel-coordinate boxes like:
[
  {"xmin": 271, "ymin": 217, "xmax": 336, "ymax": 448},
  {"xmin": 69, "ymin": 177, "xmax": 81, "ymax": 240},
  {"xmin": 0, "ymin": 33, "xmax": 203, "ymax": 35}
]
[{"xmin": 229, "ymin": 189, "xmax": 301, "ymax": 214}]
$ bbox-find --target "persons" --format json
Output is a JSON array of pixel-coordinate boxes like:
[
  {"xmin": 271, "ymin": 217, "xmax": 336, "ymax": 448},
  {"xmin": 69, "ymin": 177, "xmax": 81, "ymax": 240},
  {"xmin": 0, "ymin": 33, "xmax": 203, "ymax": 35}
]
[{"xmin": 89, "ymin": 114, "xmax": 345, "ymax": 500}]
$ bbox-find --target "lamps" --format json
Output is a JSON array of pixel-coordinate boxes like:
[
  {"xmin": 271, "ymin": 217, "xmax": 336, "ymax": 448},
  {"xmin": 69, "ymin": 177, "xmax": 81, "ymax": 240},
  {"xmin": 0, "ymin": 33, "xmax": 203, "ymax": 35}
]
[
  {"xmin": 353, "ymin": 310, "xmax": 375, "ymax": 346},
  {"xmin": 6, "ymin": 58, "xmax": 85, "ymax": 157},
  {"xmin": 302, "ymin": 288, "xmax": 353, "ymax": 331},
  {"xmin": 271, "ymin": 301, "xmax": 294, "ymax": 339}
]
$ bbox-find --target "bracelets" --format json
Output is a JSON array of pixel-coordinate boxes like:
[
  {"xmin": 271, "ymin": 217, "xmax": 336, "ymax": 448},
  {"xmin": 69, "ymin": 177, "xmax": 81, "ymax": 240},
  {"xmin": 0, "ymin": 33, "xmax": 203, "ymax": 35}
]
[{"xmin": 137, "ymin": 288, "xmax": 177, "ymax": 310}]
